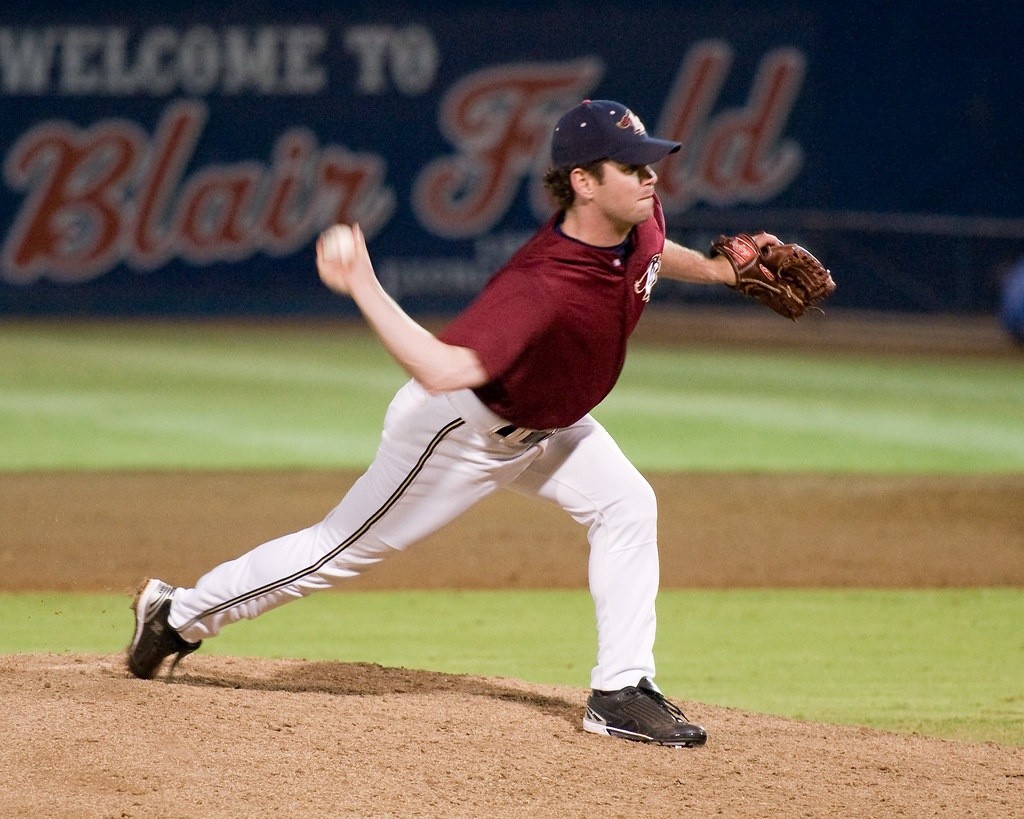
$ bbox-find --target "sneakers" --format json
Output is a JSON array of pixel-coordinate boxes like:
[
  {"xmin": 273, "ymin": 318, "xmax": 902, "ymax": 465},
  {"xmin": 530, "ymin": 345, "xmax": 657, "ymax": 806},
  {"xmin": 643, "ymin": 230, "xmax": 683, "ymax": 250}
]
[
  {"xmin": 128, "ymin": 579, "xmax": 203, "ymax": 680},
  {"xmin": 582, "ymin": 676, "xmax": 707, "ymax": 749}
]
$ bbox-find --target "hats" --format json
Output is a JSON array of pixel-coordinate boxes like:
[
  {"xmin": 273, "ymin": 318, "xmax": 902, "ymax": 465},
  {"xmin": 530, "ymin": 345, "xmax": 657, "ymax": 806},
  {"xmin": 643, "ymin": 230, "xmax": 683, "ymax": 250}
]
[{"xmin": 551, "ymin": 100, "xmax": 682, "ymax": 164}]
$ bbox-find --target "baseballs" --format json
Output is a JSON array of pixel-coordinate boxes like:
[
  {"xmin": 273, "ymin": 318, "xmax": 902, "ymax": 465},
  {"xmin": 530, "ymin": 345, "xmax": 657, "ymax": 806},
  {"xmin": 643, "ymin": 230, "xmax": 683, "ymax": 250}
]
[{"xmin": 319, "ymin": 223, "xmax": 355, "ymax": 266}]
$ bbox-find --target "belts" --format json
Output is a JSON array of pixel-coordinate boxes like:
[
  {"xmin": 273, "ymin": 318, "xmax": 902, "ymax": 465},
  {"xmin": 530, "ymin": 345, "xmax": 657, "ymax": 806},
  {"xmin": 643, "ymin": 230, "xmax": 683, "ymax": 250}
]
[{"xmin": 495, "ymin": 425, "xmax": 557, "ymax": 444}]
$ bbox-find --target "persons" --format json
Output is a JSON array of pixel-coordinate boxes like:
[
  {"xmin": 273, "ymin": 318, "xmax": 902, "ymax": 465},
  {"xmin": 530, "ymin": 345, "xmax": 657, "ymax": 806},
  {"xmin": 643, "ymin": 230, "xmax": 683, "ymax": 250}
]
[{"xmin": 128, "ymin": 101, "xmax": 786, "ymax": 746}]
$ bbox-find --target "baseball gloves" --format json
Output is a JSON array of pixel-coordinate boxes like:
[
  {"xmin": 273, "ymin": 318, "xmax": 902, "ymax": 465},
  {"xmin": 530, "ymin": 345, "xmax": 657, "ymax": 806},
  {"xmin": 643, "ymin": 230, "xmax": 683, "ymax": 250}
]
[{"xmin": 710, "ymin": 231, "xmax": 838, "ymax": 324}]
